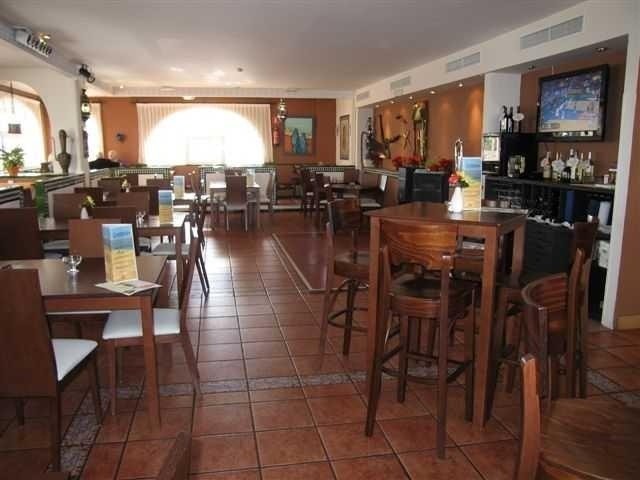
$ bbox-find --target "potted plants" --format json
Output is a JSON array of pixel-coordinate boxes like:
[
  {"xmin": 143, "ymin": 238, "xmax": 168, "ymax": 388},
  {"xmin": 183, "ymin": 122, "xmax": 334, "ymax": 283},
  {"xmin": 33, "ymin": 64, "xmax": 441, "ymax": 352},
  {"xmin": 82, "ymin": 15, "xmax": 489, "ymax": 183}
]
[{"xmin": 0, "ymin": 147, "xmax": 25, "ymax": 177}]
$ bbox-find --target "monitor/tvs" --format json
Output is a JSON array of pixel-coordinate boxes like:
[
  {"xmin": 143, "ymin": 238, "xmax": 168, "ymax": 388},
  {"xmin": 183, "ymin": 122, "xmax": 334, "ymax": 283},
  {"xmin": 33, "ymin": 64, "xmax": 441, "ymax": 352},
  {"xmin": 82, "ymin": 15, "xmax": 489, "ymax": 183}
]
[{"xmin": 536, "ymin": 64, "xmax": 609, "ymax": 142}]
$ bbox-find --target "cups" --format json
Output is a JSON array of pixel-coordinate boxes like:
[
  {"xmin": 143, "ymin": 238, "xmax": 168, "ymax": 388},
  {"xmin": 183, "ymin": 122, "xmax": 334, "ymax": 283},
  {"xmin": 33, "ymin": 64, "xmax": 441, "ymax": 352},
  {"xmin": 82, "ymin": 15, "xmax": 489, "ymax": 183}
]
[{"xmin": 609, "ymin": 169, "xmax": 616, "ymax": 185}]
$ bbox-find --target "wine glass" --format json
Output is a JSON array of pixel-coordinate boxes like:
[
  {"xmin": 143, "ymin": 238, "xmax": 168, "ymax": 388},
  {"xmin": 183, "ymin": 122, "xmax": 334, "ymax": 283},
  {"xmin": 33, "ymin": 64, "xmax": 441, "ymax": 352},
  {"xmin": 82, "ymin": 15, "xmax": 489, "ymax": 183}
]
[
  {"xmin": 62, "ymin": 255, "xmax": 82, "ymax": 273},
  {"xmin": 135, "ymin": 210, "xmax": 147, "ymax": 225}
]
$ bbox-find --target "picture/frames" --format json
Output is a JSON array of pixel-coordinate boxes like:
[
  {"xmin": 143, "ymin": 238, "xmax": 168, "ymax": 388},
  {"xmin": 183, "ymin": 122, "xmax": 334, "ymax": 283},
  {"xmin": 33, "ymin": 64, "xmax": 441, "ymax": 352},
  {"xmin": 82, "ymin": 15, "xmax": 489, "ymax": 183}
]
[
  {"xmin": 282, "ymin": 113, "xmax": 315, "ymax": 156},
  {"xmin": 339, "ymin": 114, "xmax": 350, "ymax": 160}
]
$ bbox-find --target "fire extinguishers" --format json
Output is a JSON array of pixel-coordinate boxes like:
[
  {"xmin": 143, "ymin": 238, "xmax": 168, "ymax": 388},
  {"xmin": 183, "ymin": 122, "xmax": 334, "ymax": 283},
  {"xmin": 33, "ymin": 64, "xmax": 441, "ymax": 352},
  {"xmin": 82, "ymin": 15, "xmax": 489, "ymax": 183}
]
[{"xmin": 272, "ymin": 113, "xmax": 281, "ymax": 145}]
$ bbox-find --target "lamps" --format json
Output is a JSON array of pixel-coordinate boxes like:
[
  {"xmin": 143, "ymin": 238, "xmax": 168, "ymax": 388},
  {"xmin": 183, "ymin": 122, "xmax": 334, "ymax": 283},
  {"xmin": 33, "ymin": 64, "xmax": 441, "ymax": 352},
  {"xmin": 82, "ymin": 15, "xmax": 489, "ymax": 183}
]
[
  {"xmin": 80, "ymin": 88, "xmax": 90, "ymax": 122},
  {"xmin": 62, "ymin": 59, "xmax": 97, "ymax": 86},
  {"xmin": 16, "ymin": 29, "xmax": 53, "ymax": 58},
  {"xmin": 277, "ymin": 97, "xmax": 288, "ymax": 121}
]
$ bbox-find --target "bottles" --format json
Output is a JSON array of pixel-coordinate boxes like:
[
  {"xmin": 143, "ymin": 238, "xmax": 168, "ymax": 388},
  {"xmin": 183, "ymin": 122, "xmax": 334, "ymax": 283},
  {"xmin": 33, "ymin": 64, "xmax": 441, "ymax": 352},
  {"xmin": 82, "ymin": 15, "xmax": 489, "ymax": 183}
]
[
  {"xmin": 508, "ymin": 106, "xmax": 514, "ymax": 133},
  {"xmin": 500, "ymin": 107, "xmax": 508, "ymax": 133},
  {"xmin": 539, "ymin": 148, "xmax": 595, "ymax": 183},
  {"xmin": 496, "ymin": 190, "xmax": 522, "ymax": 209},
  {"xmin": 604, "ymin": 175, "xmax": 609, "ymax": 185},
  {"xmin": 514, "ymin": 105, "xmax": 521, "ymax": 134}
]
